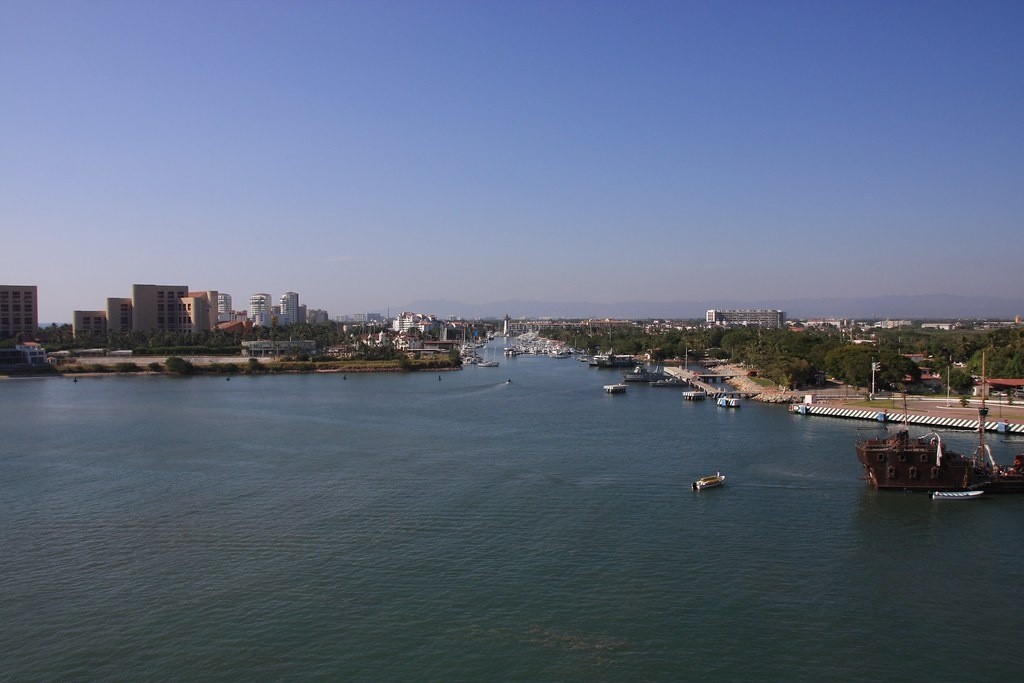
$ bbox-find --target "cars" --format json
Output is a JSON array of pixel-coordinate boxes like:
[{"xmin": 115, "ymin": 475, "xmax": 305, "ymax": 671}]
[{"xmin": 990, "ymin": 391, "xmax": 1008, "ymax": 396}]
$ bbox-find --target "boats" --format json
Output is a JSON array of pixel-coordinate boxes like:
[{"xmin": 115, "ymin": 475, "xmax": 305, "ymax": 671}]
[
  {"xmin": 691, "ymin": 471, "xmax": 726, "ymax": 490},
  {"xmin": 603, "ymin": 384, "xmax": 630, "ymax": 393},
  {"xmin": 932, "ymin": 490, "xmax": 985, "ymax": 501}
]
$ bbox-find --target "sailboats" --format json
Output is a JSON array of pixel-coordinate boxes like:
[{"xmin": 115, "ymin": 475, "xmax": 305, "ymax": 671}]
[
  {"xmin": 458, "ymin": 328, "xmax": 588, "ymax": 367},
  {"xmin": 854, "ymin": 348, "xmax": 1024, "ymax": 493}
]
